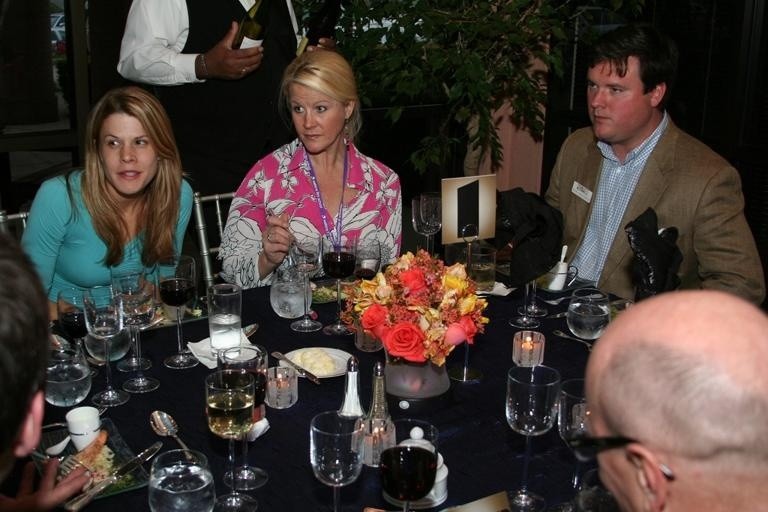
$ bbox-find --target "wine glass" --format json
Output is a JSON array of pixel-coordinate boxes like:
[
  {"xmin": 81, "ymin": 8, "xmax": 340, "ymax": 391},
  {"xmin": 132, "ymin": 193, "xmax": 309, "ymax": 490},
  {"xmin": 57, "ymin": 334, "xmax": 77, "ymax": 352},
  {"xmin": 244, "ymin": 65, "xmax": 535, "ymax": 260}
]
[
  {"xmin": 55, "ymin": 255, "xmax": 198, "ymax": 410},
  {"xmin": 349, "ymin": 237, "xmax": 382, "ymax": 353},
  {"xmin": 410, "ymin": 192, "xmax": 442, "ymax": 257},
  {"xmin": 321, "ymin": 231, "xmax": 356, "ymax": 336},
  {"xmin": 559, "ymin": 379, "xmax": 610, "ymax": 511},
  {"xmin": 307, "ymin": 412, "xmax": 361, "ymax": 512},
  {"xmin": 203, "ymin": 368, "xmax": 258, "ymax": 511},
  {"xmin": 217, "ymin": 345, "xmax": 271, "ymax": 490},
  {"xmin": 286, "ymin": 232, "xmax": 322, "ymax": 332},
  {"xmin": 379, "ymin": 421, "xmax": 439, "ymax": 512},
  {"xmin": 506, "ymin": 366, "xmax": 560, "ymax": 512}
]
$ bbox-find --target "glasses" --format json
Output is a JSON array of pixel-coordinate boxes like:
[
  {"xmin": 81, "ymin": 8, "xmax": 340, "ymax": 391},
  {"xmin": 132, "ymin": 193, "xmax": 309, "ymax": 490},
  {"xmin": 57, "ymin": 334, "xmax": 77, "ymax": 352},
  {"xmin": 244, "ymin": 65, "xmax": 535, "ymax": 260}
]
[{"xmin": 570, "ymin": 403, "xmax": 679, "ymax": 483}]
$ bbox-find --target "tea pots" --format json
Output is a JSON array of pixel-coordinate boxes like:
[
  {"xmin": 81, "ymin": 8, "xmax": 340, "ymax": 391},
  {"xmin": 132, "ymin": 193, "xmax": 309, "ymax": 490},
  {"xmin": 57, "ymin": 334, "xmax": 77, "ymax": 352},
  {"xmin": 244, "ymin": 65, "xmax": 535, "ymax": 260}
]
[{"xmin": 270, "ymin": 261, "xmax": 313, "ymax": 320}]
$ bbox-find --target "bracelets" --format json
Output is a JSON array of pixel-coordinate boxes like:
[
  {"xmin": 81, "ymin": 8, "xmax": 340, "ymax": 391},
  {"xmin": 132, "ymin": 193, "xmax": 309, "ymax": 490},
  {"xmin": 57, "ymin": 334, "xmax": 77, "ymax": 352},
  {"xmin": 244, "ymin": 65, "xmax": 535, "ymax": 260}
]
[{"xmin": 198, "ymin": 54, "xmax": 209, "ymax": 79}]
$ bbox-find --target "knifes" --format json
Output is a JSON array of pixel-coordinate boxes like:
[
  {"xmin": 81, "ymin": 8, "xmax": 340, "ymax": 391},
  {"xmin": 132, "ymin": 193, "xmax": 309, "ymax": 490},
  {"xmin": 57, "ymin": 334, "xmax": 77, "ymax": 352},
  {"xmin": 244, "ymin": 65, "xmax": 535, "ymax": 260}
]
[
  {"xmin": 271, "ymin": 351, "xmax": 320, "ymax": 385},
  {"xmin": 66, "ymin": 442, "xmax": 162, "ymax": 511}
]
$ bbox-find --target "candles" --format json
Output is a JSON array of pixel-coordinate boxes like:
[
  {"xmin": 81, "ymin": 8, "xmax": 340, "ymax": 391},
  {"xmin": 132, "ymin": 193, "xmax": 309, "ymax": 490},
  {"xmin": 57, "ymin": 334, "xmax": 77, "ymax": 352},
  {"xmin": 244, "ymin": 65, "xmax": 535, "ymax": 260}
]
[
  {"xmin": 365, "ymin": 428, "xmax": 391, "ymax": 459},
  {"xmin": 520, "ymin": 336, "xmax": 534, "ymax": 361},
  {"xmin": 268, "ymin": 374, "xmax": 292, "ymax": 405}
]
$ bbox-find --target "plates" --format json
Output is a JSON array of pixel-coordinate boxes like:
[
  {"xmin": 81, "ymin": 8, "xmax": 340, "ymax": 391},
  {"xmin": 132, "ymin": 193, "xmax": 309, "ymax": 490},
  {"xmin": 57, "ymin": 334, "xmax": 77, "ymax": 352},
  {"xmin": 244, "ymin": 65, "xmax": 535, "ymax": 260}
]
[
  {"xmin": 277, "ymin": 345, "xmax": 355, "ymax": 381},
  {"xmin": 30, "ymin": 418, "xmax": 147, "ymax": 503}
]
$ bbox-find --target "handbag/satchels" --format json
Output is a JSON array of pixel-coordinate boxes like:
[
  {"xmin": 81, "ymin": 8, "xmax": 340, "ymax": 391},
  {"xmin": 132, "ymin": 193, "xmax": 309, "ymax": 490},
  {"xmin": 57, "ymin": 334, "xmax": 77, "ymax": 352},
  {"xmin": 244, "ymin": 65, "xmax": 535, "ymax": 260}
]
[{"xmin": 495, "ymin": 188, "xmax": 563, "ymax": 290}]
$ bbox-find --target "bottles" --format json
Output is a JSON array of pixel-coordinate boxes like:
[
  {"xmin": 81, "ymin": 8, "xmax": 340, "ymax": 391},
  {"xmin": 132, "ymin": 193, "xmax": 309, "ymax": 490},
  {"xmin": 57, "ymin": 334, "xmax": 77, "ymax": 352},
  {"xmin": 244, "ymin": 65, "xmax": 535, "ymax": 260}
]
[
  {"xmin": 364, "ymin": 362, "xmax": 397, "ymax": 471},
  {"xmin": 335, "ymin": 357, "xmax": 366, "ymax": 426}
]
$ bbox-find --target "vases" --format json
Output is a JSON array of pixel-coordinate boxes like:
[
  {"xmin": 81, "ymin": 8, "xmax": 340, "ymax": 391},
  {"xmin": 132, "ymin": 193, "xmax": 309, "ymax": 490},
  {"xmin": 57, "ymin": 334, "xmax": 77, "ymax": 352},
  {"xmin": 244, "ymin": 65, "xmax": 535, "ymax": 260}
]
[{"xmin": 383, "ymin": 346, "xmax": 450, "ymax": 398}]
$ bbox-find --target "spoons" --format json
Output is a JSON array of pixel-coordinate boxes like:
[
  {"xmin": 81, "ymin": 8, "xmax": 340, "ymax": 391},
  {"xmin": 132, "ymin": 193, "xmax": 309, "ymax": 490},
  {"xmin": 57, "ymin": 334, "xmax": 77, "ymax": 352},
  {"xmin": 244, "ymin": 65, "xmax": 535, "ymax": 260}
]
[
  {"xmin": 150, "ymin": 410, "xmax": 195, "ymax": 462},
  {"xmin": 182, "ymin": 323, "xmax": 259, "ymax": 353}
]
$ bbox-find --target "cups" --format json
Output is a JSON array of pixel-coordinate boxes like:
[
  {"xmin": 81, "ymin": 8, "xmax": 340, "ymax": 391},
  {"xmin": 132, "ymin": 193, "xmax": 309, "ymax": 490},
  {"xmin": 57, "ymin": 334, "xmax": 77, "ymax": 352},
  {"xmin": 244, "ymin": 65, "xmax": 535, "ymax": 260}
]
[
  {"xmin": 568, "ymin": 288, "xmax": 613, "ymax": 341},
  {"xmin": 508, "ymin": 280, "xmax": 547, "ymax": 331},
  {"xmin": 41, "ymin": 343, "xmax": 91, "ymax": 408},
  {"xmin": 540, "ymin": 267, "xmax": 580, "ymax": 305},
  {"xmin": 148, "ymin": 449, "xmax": 214, "ymax": 511},
  {"xmin": 66, "ymin": 406, "xmax": 102, "ymax": 453},
  {"xmin": 266, "ymin": 367, "xmax": 298, "ymax": 409},
  {"xmin": 204, "ymin": 283, "xmax": 242, "ymax": 350},
  {"xmin": 465, "ymin": 244, "xmax": 499, "ymax": 290}
]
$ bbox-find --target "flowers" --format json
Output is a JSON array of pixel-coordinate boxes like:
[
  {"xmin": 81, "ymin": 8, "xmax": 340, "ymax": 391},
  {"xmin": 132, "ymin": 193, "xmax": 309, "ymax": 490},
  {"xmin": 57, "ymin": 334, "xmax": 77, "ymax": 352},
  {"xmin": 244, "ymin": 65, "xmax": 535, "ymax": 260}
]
[{"xmin": 337, "ymin": 250, "xmax": 489, "ymax": 368}]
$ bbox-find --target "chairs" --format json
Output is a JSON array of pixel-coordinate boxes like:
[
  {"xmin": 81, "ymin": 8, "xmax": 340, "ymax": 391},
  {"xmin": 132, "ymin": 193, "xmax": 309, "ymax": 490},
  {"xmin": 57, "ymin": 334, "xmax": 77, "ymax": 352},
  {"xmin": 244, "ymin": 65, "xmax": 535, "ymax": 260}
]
[
  {"xmin": 0, "ymin": 211, "xmax": 29, "ymax": 240},
  {"xmin": 194, "ymin": 192, "xmax": 235, "ymax": 286}
]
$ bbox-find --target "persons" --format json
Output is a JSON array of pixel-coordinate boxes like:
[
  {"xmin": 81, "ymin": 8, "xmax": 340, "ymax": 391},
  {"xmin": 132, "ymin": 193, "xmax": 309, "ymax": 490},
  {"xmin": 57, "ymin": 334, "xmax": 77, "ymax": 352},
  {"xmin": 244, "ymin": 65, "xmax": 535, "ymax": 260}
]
[
  {"xmin": 0, "ymin": 228, "xmax": 93, "ymax": 512},
  {"xmin": 540, "ymin": 22, "xmax": 767, "ymax": 308},
  {"xmin": 116, "ymin": 0, "xmax": 337, "ymax": 294},
  {"xmin": 19, "ymin": 85, "xmax": 194, "ymax": 322},
  {"xmin": 214, "ymin": 49, "xmax": 405, "ymax": 291},
  {"xmin": 581, "ymin": 287, "xmax": 768, "ymax": 511}
]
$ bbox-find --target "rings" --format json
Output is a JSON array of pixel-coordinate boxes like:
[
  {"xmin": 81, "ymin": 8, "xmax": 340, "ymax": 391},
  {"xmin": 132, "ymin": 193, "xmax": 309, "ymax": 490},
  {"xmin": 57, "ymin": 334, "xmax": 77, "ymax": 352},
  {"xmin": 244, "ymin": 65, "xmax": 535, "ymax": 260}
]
[
  {"xmin": 267, "ymin": 233, "xmax": 271, "ymax": 241},
  {"xmin": 239, "ymin": 66, "xmax": 248, "ymax": 75}
]
